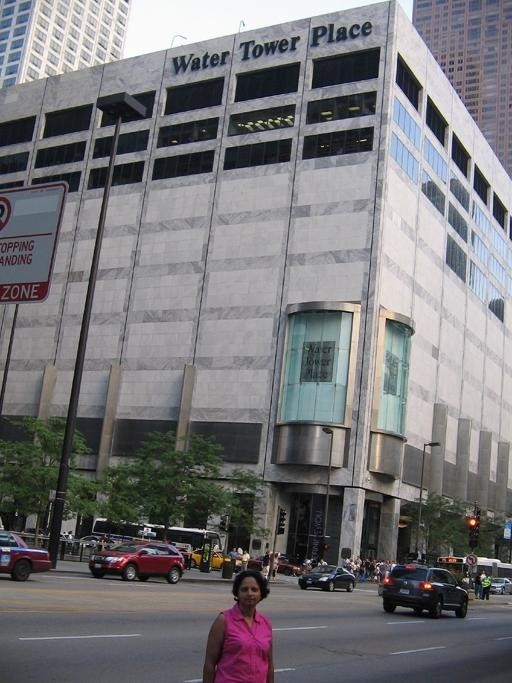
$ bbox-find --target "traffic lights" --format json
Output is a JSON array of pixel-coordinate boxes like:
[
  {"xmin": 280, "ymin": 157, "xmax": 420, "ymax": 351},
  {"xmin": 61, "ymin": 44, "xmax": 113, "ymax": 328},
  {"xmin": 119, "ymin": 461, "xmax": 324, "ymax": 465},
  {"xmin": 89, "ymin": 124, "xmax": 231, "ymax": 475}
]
[
  {"xmin": 275, "ymin": 507, "xmax": 287, "ymax": 534},
  {"xmin": 324, "ymin": 543, "xmax": 328, "ymax": 549},
  {"xmin": 219, "ymin": 514, "xmax": 230, "ymax": 531},
  {"xmin": 468, "ymin": 517, "xmax": 480, "ymax": 547}
]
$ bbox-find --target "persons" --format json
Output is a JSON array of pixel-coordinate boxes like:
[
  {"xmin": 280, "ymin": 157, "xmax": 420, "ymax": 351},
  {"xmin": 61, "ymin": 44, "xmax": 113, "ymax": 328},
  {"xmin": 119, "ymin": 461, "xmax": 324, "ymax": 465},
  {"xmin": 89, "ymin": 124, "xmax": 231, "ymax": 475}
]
[
  {"xmin": 474, "ymin": 571, "xmax": 482, "ymax": 599},
  {"xmin": 262, "ymin": 550, "xmax": 271, "ymax": 579},
  {"xmin": 271, "ymin": 551, "xmax": 281, "ymax": 580},
  {"xmin": 228, "ymin": 545, "xmax": 239, "ymax": 574},
  {"xmin": 67, "ymin": 529, "xmax": 72, "ymax": 554},
  {"xmin": 318, "ymin": 559, "xmax": 328, "ymax": 571},
  {"xmin": 301, "ymin": 558, "xmax": 313, "ymax": 571},
  {"xmin": 201, "ymin": 567, "xmax": 274, "ymax": 682},
  {"xmin": 89, "ymin": 536, "xmax": 97, "ymax": 555},
  {"xmin": 239, "ymin": 549, "xmax": 250, "ymax": 571},
  {"xmin": 62, "ymin": 530, "xmax": 65, "ymax": 539},
  {"xmin": 342, "ymin": 555, "xmax": 399, "ymax": 583},
  {"xmin": 479, "ymin": 569, "xmax": 487, "ymax": 599},
  {"xmin": 481, "ymin": 574, "xmax": 491, "ymax": 600}
]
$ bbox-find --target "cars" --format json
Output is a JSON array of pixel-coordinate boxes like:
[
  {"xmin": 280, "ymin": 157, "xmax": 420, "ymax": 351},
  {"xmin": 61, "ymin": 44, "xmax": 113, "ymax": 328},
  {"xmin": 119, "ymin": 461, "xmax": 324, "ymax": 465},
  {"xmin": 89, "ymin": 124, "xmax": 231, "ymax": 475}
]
[
  {"xmin": 79, "ymin": 534, "xmax": 115, "ymax": 549},
  {"xmin": 186, "ymin": 548, "xmax": 242, "ymax": 571},
  {"xmin": 298, "ymin": 563, "xmax": 357, "ymax": 592},
  {"xmin": 20, "ymin": 526, "xmax": 50, "ymax": 545},
  {"xmin": 246, "ymin": 551, "xmax": 302, "ymax": 576},
  {"xmin": 489, "ymin": 577, "xmax": 511, "ymax": 594},
  {"xmin": 1, "ymin": 529, "xmax": 53, "ymax": 582}
]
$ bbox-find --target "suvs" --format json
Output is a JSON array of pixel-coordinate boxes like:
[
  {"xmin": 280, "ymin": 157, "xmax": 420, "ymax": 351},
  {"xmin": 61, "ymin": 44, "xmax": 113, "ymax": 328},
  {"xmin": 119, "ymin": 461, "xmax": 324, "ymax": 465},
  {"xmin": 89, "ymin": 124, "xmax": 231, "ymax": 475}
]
[
  {"xmin": 89, "ymin": 539, "xmax": 185, "ymax": 584},
  {"xmin": 377, "ymin": 563, "xmax": 469, "ymax": 618}
]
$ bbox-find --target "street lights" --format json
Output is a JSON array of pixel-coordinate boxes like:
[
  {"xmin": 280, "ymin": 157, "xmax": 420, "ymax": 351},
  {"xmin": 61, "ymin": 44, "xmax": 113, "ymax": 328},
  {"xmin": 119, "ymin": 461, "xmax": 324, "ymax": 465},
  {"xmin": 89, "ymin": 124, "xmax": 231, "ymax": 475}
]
[
  {"xmin": 415, "ymin": 441, "xmax": 441, "ymax": 553},
  {"xmin": 320, "ymin": 426, "xmax": 333, "ymax": 562},
  {"xmin": 46, "ymin": 91, "xmax": 148, "ymax": 568}
]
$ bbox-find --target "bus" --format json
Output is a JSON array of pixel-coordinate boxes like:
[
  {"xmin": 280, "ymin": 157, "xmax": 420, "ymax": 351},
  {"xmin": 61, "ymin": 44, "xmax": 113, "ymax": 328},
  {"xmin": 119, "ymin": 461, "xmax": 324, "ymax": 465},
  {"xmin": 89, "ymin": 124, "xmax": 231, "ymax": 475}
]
[
  {"xmin": 436, "ymin": 556, "xmax": 511, "ymax": 589},
  {"xmin": 92, "ymin": 516, "xmax": 223, "ymax": 554}
]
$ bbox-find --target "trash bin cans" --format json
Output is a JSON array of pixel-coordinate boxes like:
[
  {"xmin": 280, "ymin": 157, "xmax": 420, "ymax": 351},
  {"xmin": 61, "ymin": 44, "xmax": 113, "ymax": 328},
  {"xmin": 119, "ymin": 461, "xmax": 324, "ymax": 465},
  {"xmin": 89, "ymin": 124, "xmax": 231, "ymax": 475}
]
[{"xmin": 222, "ymin": 562, "xmax": 234, "ymax": 579}]
